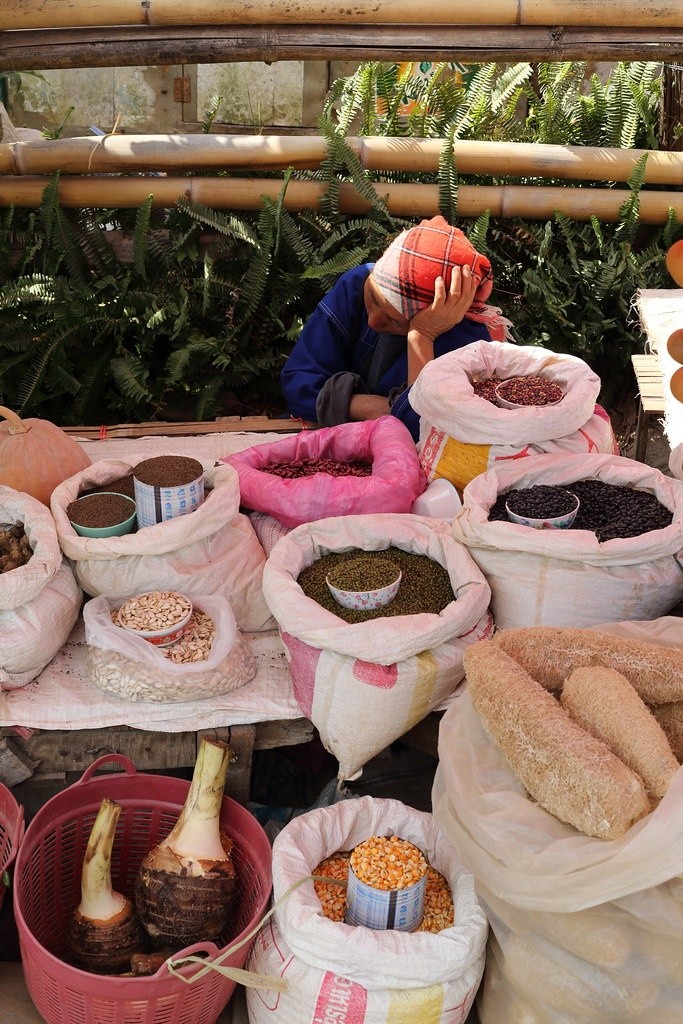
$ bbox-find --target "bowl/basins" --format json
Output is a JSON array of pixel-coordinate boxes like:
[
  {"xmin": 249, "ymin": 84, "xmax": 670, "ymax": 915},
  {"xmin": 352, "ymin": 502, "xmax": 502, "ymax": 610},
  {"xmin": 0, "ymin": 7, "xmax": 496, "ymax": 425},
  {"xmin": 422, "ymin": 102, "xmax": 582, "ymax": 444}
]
[
  {"xmin": 495, "ymin": 378, "xmax": 563, "ymax": 411},
  {"xmin": 68, "ymin": 493, "xmax": 137, "ymax": 538},
  {"xmin": 410, "ymin": 478, "xmax": 462, "ymax": 521},
  {"xmin": 120, "ymin": 590, "xmax": 193, "ymax": 647},
  {"xmin": 326, "ymin": 559, "xmax": 402, "ymax": 610},
  {"xmin": 506, "ymin": 489, "xmax": 579, "ymax": 530}
]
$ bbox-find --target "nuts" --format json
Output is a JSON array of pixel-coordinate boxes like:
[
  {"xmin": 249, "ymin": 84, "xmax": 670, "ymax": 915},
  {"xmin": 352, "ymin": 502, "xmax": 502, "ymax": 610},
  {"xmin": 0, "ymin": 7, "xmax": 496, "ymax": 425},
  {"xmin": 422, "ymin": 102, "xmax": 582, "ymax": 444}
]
[{"xmin": 88, "ymin": 590, "xmax": 256, "ymax": 702}]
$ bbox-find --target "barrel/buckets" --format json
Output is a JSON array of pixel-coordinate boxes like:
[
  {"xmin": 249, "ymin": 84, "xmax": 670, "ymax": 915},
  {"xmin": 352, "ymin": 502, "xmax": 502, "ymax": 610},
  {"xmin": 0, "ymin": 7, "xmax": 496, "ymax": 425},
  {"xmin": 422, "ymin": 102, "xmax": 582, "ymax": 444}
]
[
  {"xmin": 345, "ymin": 837, "xmax": 427, "ymax": 932},
  {"xmin": 134, "ymin": 456, "xmax": 205, "ymax": 531}
]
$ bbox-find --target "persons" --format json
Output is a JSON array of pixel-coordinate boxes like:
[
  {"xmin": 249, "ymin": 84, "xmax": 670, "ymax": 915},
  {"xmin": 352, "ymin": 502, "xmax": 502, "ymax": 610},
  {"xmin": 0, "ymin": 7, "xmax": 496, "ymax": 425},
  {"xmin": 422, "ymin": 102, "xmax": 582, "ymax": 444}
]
[{"xmin": 280, "ymin": 215, "xmax": 516, "ymax": 444}]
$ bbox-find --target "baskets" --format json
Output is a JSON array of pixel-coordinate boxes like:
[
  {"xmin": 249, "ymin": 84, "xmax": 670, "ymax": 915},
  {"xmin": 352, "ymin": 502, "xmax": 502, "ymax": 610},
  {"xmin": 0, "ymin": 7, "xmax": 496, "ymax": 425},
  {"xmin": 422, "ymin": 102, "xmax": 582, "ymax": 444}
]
[
  {"xmin": 0, "ymin": 781, "xmax": 25, "ymax": 912},
  {"xmin": 13, "ymin": 753, "xmax": 274, "ymax": 1024}
]
